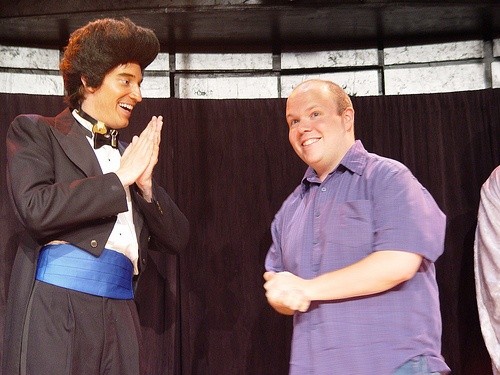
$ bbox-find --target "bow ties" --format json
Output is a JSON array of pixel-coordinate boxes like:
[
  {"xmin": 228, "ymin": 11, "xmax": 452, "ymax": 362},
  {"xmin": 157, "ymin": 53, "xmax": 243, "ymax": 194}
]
[{"xmin": 72, "ymin": 115, "xmax": 118, "ymax": 149}]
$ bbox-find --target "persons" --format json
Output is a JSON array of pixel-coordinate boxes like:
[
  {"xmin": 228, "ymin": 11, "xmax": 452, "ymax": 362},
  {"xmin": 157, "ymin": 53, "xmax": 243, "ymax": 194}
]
[
  {"xmin": 473, "ymin": 163, "xmax": 500, "ymax": 375},
  {"xmin": 0, "ymin": 17, "xmax": 191, "ymax": 375},
  {"xmin": 262, "ymin": 79, "xmax": 453, "ymax": 375}
]
